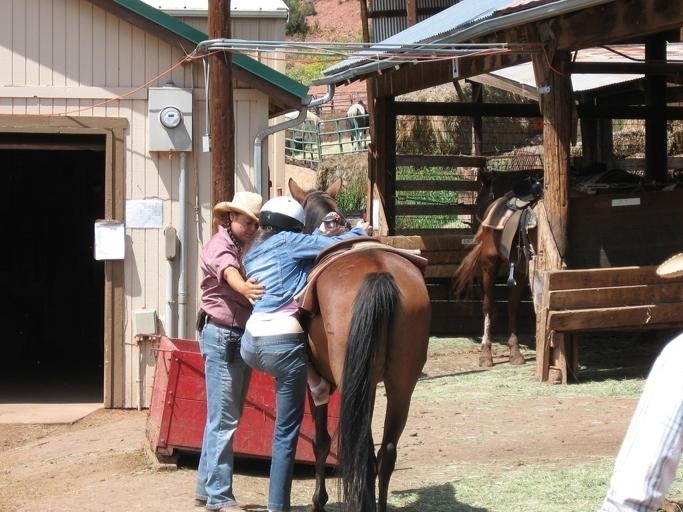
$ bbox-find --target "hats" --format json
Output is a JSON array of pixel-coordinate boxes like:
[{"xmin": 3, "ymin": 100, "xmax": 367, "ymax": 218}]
[
  {"xmin": 213, "ymin": 192, "xmax": 262, "ymax": 225},
  {"xmin": 657, "ymin": 252, "xmax": 683, "ymax": 279}
]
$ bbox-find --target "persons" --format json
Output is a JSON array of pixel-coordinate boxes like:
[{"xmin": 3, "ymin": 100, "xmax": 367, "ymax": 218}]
[
  {"xmin": 192, "ymin": 191, "xmax": 266, "ymax": 512},
  {"xmin": 594, "ymin": 249, "xmax": 683, "ymax": 512},
  {"xmin": 240, "ymin": 195, "xmax": 375, "ymax": 512}
]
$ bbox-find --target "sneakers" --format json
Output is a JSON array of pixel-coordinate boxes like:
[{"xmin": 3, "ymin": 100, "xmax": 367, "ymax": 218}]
[
  {"xmin": 311, "ymin": 380, "xmax": 331, "ymax": 406},
  {"xmin": 194, "ymin": 498, "xmax": 247, "ymax": 512}
]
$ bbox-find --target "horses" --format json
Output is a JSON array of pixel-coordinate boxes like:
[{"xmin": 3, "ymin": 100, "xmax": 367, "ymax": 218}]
[
  {"xmin": 286, "ymin": 175, "xmax": 430, "ymax": 511},
  {"xmin": 346, "ymin": 100, "xmax": 370, "ymax": 149},
  {"xmin": 285, "ymin": 110, "xmax": 325, "ymax": 130},
  {"xmin": 449, "ymin": 160, "xmax": 683, "ymax": 368}
]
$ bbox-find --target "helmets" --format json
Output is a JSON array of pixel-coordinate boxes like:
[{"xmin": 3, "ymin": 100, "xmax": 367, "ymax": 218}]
[{"xmin": 260, "ymin": 196, "xmax": 305, "ymax": 233}]
